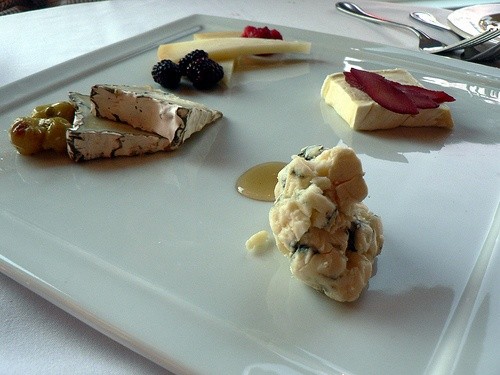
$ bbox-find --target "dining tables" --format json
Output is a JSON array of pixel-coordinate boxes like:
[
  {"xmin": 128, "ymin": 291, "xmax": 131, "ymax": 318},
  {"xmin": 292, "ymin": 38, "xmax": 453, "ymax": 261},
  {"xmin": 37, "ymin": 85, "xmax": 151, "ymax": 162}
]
[{"xmin": 0, "ymin": 0, "xmax": 463, "ymax": 375}]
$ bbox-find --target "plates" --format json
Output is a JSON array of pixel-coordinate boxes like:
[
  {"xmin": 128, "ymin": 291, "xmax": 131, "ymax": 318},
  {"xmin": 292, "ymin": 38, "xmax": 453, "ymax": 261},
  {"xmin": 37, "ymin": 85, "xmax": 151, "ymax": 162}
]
[
  {"xmin": 446, "ymin": 4, "xmax": 500, "ymax": 60},
  {"xmin": 0, "ymin": 14, "xmax": 500, "ymax": 375}
]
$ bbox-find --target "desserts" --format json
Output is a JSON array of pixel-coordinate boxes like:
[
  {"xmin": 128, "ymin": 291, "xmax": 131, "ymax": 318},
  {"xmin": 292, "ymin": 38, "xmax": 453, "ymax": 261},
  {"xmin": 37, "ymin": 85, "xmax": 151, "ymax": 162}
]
[
  {"xmin": 9, "ymin": 82, "xmax": 225, "ymax": 164},
  {"xmin": 320, "ymin": 67, "xmax": 456, "ymax": 130},
  {"xmin": 246, "ymin": 140, "xmax": 385, "ymax": 304},
  {"xmin": 151, "ymin": 24, "xmax": 313, "ymax": 90}
]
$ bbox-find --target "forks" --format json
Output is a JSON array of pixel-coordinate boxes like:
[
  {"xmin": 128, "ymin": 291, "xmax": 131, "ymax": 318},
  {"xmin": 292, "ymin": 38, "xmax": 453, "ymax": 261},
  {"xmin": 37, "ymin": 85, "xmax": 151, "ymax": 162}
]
[
  {"xmin": 410, "ymin": 11, "xmax": 500, "ymax": 65},
  {"xmin": 336, "ymin": 2, "xmax": 500, "ymax": 56}
]
